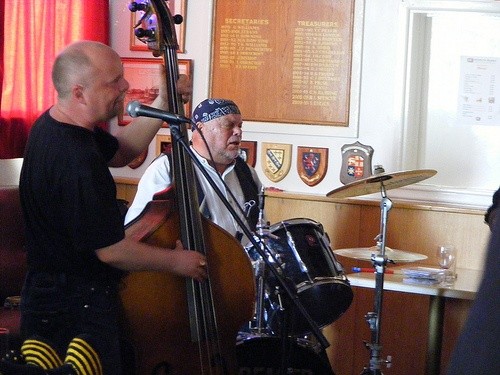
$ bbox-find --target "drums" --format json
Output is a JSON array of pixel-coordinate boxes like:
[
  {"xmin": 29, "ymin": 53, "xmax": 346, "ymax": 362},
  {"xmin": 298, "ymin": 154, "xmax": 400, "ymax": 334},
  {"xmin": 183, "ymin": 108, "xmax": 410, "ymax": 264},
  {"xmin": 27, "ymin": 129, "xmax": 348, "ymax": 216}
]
[
  {"xmin": 246, "ymin": 218, "xmax": 354, "ymax": 334},
  {"xmin": 234, "ymin": 330, "xmax": 334, "ymax": 375}
]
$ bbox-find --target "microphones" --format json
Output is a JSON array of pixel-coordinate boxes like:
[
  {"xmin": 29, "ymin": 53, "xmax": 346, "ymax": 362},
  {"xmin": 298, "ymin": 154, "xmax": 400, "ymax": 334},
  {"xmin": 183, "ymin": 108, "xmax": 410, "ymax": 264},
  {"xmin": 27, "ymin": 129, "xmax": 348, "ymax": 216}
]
[{"xmin": 126, "ymin": 101, "xmax": 192, "ymax": 124}]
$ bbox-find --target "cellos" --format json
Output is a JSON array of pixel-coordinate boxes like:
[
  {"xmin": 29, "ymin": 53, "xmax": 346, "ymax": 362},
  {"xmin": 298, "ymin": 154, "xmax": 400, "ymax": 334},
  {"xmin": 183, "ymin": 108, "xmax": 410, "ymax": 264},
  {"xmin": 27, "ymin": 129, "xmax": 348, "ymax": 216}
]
[{"xmin": 112, "ymin": 0, "xmax": 256, "ymax": 375}]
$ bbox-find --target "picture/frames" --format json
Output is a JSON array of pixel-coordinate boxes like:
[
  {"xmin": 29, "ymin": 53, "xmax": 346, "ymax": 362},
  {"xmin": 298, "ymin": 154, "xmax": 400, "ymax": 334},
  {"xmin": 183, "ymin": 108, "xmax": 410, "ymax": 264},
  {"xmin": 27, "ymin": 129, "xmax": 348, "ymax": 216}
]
[
  {"xmin": 128, "ymin": 0, "xmax": 186, "ymax": 54},
  {"xmin": 117, "ymin": 55, "xmax": 195, "ymax": 131}
]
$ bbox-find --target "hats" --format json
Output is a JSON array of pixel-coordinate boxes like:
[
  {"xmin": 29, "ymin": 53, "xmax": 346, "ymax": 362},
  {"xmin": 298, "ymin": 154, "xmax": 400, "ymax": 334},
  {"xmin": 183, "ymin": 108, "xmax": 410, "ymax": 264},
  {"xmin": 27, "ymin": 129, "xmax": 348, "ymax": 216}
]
[{"xmin": 191, "ymin": 98, "xmax": 241, "ymax": 131}]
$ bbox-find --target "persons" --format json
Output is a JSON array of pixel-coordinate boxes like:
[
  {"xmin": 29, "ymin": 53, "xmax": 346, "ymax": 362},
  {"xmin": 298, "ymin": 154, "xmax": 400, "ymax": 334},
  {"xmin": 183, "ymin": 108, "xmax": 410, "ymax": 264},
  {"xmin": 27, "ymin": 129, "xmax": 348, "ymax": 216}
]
[
  {"xmin": 18, "ymin": 41, "xmax": 211, "ymax": 375},
  {"xmin": 124, "ymin": 98, "xmax": 263, "ymax": 249},
  {"xmin": 451, "ymin": 186, "xmax": 500, "ymax": 375}
]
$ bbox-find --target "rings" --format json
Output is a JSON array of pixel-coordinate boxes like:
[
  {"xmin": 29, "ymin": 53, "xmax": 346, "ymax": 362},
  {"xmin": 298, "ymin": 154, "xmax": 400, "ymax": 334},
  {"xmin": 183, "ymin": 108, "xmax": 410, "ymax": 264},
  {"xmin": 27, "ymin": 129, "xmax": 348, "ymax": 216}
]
[{"xmin": 198, "ymin": 260, "xmax": 206, "ymax": 266}]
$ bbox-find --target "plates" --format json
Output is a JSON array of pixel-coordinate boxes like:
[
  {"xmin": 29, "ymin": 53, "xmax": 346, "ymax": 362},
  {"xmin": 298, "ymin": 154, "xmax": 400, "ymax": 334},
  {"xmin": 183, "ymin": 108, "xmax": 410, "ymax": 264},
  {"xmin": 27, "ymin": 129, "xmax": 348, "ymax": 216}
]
[{"xmin": 401, "ymin": 267, "xmax": 447, "ymax": 280}]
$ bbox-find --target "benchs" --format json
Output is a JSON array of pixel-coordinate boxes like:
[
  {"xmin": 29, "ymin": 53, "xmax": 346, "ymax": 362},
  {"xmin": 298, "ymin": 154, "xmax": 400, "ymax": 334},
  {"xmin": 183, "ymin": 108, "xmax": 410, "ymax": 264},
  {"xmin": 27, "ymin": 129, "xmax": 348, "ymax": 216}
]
[{"xmin": 0, "ymin": 184, "xmax": 31, "ymax": 339}]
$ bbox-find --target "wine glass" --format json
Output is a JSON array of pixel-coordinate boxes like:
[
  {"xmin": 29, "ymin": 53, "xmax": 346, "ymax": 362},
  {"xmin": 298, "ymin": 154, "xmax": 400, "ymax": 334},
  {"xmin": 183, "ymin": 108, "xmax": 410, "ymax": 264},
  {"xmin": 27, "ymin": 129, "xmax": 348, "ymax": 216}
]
[{"xmin": 435, "ymin": 245, "xmax": 457, "ymax": 286}]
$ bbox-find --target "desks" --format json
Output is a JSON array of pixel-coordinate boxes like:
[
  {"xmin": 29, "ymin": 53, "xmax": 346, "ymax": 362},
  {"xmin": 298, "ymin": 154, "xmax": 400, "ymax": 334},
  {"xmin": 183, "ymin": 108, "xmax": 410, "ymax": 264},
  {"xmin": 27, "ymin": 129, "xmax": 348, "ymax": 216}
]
[{"xmin": 347, "ymin": 265, "xmax": 482, "ymax": 375}]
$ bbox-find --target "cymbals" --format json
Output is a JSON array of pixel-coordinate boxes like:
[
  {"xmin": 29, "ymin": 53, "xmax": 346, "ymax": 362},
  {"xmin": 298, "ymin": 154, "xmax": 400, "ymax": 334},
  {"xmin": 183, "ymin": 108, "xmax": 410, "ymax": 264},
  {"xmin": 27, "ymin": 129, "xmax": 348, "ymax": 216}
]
[
  {"xmin": 330, "ymin": 244, "xmax": 428, "ymax": 264},
  {"xmin": 325, "ymin": 169, "xmax": 436, "ymax": 199}
]
